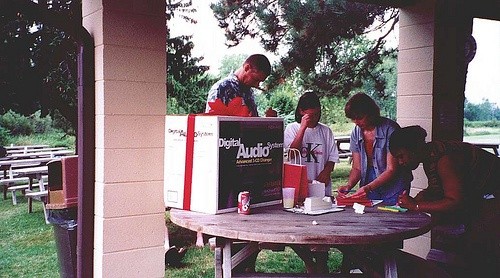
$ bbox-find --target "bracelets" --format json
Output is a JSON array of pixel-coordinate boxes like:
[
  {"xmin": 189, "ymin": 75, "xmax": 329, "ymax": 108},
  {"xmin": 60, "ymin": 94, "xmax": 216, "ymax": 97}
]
[{"xmin": 417, "ymin": 202, "xmax": 419, "ymax": 211}]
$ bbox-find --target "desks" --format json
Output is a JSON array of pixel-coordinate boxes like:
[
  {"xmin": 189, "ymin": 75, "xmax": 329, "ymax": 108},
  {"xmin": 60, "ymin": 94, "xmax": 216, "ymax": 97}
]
[
  {"xmin": 24, "ymin": 190, "xmax": 48, "ymax": 202},
  {"xmin": 11, "ymin": 167, "xmax": 51, "ymax": 226},
  {"xmin": 0, "ymin": 157, "xmax": 59, "ymax": 199},
  {"xmin": 6, "ymin": 146, "xmax": 68, "ymax": 154},
  {"xmin": 167, "ymin": 202, "xmax": 436, "ymax": 278},
  {"xmin": 11, "ymin": 151, "xmax": 74, "ymax": 159},
  {"xmin": 3, "ymin": 144, "xmax": 50, "ymax": 150},
  {"xmin": 334, "ymin": 136, "xmax": 353, "ymax": 165}
]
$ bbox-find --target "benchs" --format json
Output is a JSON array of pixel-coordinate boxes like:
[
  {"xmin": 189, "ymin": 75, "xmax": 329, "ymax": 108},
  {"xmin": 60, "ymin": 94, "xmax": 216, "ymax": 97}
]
[
  {"xmin": 337, "ymin": 152, "xmax": 353, "ymax": 159},
  {"xmin": 0, "ymin": 174, "xmax": 48, "ymax": 186},
  {"xmin": 6, "ymin": 181, "xmax": 47, "ymax": 196}
]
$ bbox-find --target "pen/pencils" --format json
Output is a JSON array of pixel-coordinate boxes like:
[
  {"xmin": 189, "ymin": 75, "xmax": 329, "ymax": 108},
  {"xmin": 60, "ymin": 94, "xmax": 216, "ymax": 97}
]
[
  {"xmin": 377, "ymin": 206, "xmax": 400, "ymax": 212},
  {"xmin": 333, "ymin": 190, "xmax": 356, "ymax": 192}
]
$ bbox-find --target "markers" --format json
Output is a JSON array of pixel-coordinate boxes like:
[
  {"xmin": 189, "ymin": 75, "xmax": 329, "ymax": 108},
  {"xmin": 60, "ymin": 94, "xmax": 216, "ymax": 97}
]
[{"xmin": 395, "ymin": 190, "xmax": 407, "ymax": 206}]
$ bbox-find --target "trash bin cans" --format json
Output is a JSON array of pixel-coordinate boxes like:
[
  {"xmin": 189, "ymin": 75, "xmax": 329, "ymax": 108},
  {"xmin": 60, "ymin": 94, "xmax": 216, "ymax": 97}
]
[{"xmin": 46, "ymin": 156, "xmax": 78, "ymax": 278}]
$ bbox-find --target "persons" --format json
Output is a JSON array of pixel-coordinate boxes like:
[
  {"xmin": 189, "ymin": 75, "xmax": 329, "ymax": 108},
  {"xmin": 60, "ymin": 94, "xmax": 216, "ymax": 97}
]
[
  {"xmin": 388, "ymin": 125, "xmax": 500, "ymax": 259},
  {"xmin": 284, "ymin": 92, "xmax": 339, "ymax": 194},
  {"xmin": 337, "ymin": 92, "xmax": 414, "ymax": 205},
  {"xmin": 206, "ymin": 54, "xmax": 277, "ymax": 118}
]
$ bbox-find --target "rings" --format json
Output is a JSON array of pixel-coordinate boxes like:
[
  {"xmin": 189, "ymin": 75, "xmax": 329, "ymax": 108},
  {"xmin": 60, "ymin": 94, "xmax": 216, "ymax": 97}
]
[{"xmin": 400, "ymin": 201, "xmax": 402, "ymax": 204}]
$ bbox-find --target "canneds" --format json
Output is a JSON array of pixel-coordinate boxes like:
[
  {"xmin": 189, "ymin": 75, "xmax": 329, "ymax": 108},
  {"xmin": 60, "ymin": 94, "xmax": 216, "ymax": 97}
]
[{"xmin": 238, "ymin": 191, "xmax": 250, "ymax": 215}]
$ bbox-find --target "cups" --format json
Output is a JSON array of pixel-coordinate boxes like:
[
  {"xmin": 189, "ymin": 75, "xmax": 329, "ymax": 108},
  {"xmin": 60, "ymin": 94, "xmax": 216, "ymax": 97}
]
[{"xmin": 282, "ymin": 188, "xmax": 295, "ymax": 208}]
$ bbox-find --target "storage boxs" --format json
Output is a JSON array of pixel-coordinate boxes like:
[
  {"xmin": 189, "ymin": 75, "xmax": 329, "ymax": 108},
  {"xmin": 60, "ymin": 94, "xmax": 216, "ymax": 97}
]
[{"xmin": 163, "ymin": 114, "xmax": 287, "ymax": 214}]
